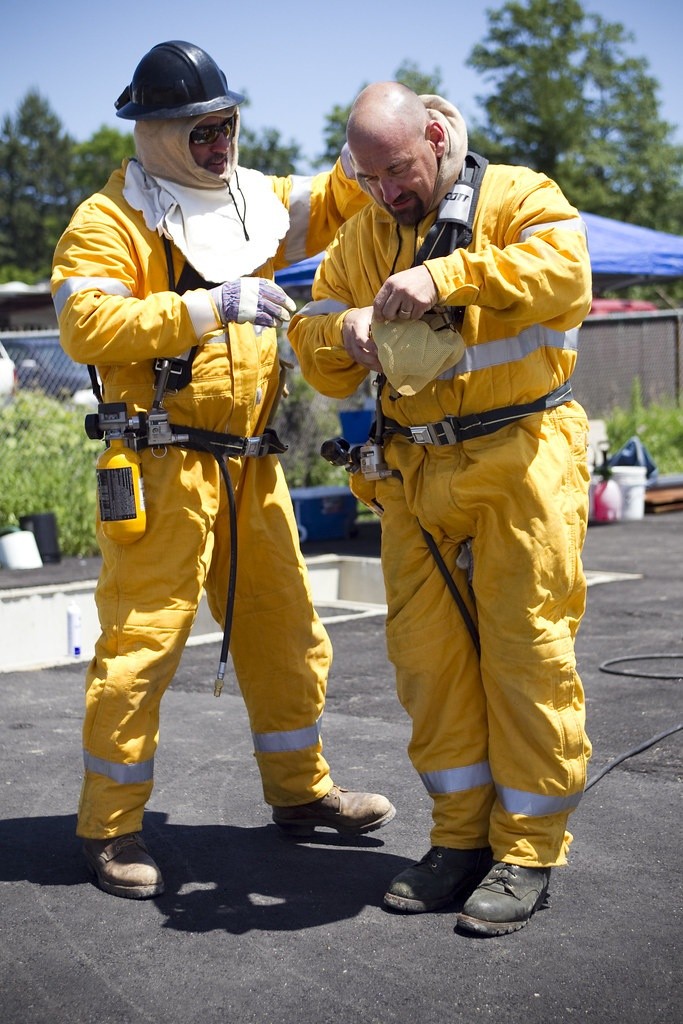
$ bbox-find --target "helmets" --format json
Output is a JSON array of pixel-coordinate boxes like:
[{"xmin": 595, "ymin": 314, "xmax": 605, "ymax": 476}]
[{"xmin": 115, "ymin": 40, "xmax": 245, "ymax": 121}]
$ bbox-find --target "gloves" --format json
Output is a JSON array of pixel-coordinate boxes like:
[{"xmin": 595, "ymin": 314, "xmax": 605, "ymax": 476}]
[
  {"xmin": 340, "ymin": 142, "xmax": 358, "ymax": 180},
  {"xmin": 222, "ymin": 277, "xmax": 296, "ymax": 328}
]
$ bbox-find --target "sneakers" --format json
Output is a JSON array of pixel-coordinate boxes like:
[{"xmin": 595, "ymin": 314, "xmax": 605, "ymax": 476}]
[
  {"xmin": 272, "ymin": 786, "xmax": 397, "ymax": 836},
  {"xmin": 384, "ymin": 847, "xmax": 492, "ymax": 912},
  {"xmin": 81, "ymin": 832, "xmax": 165, "ymax": 898},
  {"xmin": 456, "ymin": 858, "xmax": 550, "ymax": 936}
]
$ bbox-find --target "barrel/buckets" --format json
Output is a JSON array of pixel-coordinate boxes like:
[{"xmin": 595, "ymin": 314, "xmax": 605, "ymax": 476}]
[
  {"xmin": 611, "ymin": 466, "xmax": 647, "ymax": 520},
  {"xmin": 19, "ymin": 514, "xmax": 61, "ymax": 565}
]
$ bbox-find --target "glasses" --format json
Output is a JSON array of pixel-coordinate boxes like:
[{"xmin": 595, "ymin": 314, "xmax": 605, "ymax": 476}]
[{"xmin": 190, "ymin": 115, "xmax": 237, "ymax": 145}]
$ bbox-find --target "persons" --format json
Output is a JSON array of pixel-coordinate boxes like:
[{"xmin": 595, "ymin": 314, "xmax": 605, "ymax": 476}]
[
  {"xmin": 50, "ymin": 41, "xmax": 398, "ymax": 899},
  {"xmin": 286, "ymin": 81, "xmax": 592, "ymax": 934}
]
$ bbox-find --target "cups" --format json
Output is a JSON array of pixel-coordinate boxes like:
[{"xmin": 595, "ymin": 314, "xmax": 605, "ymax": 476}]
[{"xmin": 339, "ymin": 410, "xmax": 373, "ymax": 445}]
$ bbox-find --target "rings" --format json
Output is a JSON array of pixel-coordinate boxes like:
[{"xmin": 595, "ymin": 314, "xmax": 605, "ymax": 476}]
[{"xmin": 400, "ymin": 309, "xmax": 411, "ymax": 315}]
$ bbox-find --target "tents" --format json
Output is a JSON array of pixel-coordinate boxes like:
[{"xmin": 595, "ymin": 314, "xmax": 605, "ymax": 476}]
[{"xmin": 273, "ymin": 212, "xmax": 683, "ymax": 302}]
